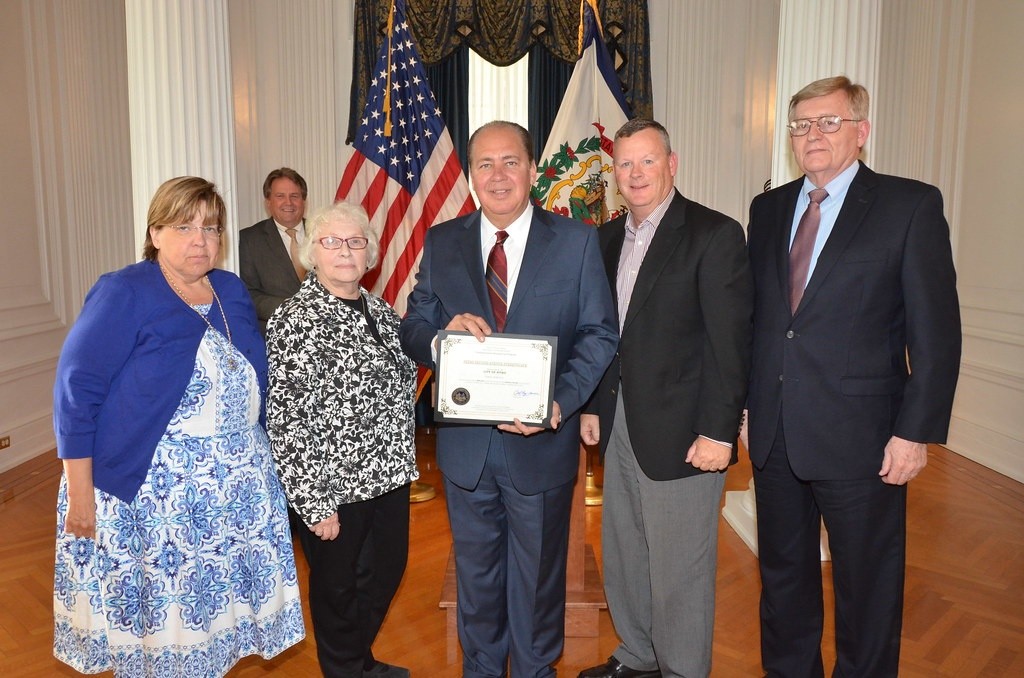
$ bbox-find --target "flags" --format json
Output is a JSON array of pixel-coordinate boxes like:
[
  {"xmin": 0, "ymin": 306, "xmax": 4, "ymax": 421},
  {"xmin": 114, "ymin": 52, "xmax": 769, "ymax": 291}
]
[
  {"xmin": 529, "ymin": 0, "xmax": 652, "ymax": 225},
  {"xmin": 331, "ymin": 0, "xmax": 477, "ymax": 404}
]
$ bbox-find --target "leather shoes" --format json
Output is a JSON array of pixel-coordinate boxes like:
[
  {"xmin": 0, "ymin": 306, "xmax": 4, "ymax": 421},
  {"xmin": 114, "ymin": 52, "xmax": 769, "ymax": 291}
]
[{"xmin": 577, "ymin": 655, "xmax": 663, "ymax": 678}]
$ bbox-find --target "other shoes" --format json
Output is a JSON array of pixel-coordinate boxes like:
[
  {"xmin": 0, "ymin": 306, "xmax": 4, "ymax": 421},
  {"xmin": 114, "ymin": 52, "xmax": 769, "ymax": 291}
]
[{"xmin": 363, "ymin": 660, "xmax": 410, "ymax": 678}]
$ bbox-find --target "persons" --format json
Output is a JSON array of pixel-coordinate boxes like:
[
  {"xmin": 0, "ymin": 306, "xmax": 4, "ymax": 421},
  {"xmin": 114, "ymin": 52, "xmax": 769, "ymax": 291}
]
[
  {"xmin": 739, "ymin": 76, "xmax": 961, "ymax": 678},
  {"xmin": 265, "ymin": 200, "xmax": 420, "ymax": 678},
  {"xmin": 240, "ymin": 167, "xmax": 308, "ymax": 341},
  {"xmin": 397, "ymin": 120, "xmax": 620, "ymax": 678},
  {"xmin": 52, "ymin": 176, "xmax": 306, "ymax": 678},
  {"xmin": 577, "ymin": 119, "xmax": 753, "ymax": 678}
]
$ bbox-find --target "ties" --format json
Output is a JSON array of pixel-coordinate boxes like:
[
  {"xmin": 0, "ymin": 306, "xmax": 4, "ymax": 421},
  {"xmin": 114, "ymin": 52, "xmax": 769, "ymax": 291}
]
[
  {"xmin": 486, "ymin": 231, "xmax": 509, "ymax": 333},
  {"xmin": 789, "ymin": 188, "xmax": 829, "ymax": 318},
  {"xmin": 285, "ymin": 229, "xmax": 308, "ymax": 284}
]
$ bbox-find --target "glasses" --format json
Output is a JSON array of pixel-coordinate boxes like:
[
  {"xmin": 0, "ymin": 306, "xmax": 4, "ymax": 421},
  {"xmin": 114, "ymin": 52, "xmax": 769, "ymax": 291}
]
[
  {"xmin": 312, "ymin": 236, "xmax": 368, "ymax": 250},
  {"xmin": 166, "ymin": 224, "xmax": 222, "ymax": 241}
]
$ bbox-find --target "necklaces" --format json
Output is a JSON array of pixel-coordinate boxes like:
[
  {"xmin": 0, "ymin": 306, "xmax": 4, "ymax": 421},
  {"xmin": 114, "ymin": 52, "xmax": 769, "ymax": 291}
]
[{"xmin": 160, "ymin": 265, "xmax": 234, "ymax": 369}]
